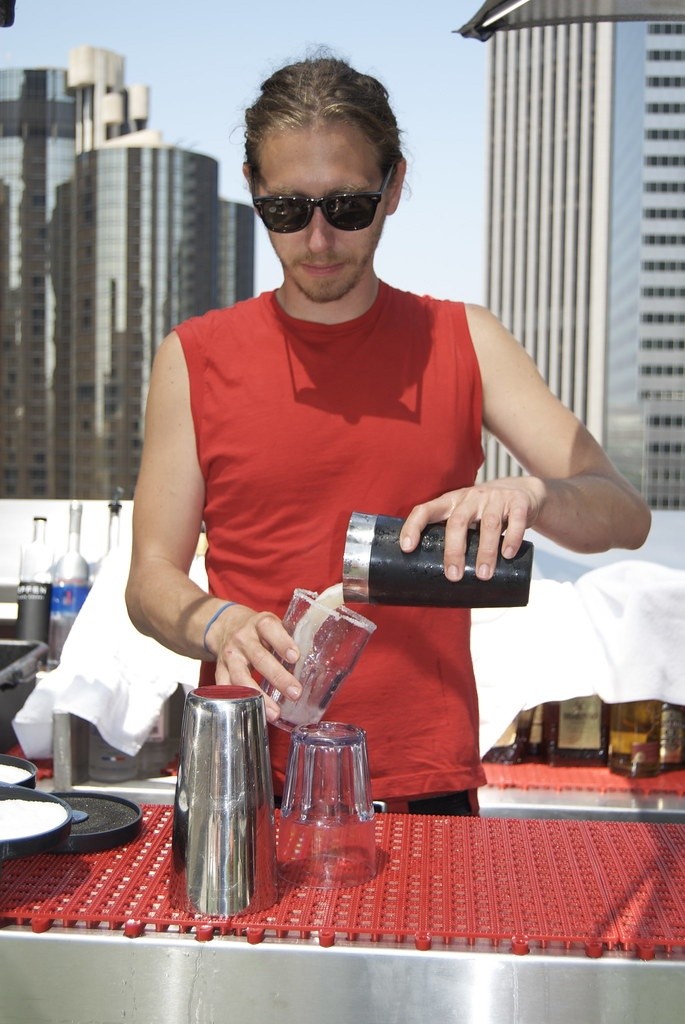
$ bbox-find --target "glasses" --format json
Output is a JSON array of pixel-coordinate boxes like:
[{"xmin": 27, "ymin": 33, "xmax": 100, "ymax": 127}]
[{"xmin": 252, "ymin": 161, "xmax": 395, "ymax": 233}]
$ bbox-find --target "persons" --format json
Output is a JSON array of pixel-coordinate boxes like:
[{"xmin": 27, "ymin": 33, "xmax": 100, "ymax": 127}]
[{"xmin": 125, "ymin": 59, "xmax": 652, "ymax": 817}]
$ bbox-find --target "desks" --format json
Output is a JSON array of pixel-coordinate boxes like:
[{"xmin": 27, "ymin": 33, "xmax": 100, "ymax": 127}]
[
  {"xmin": 478, "ymin": 786, "xmax": 685, "ymax": 824},
  {"xmin": 1, "ymin": 925, "xmax": 685, "ymax": 1024}
]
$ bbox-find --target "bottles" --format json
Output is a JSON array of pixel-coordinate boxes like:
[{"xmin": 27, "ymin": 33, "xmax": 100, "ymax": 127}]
[
  {"xmin": 89, "ymin": 496, "xmax": 123, "ymax": 588},
  {"xmin": 47, "ymin": 503, "xmax": 89, "ymax": 666},
  {"xmin": 483, "ymin": 695, "xmax": 685, "ymax": 778},
  {"xmin": 16, "ymin": 517, "xmax": 52, "ymax": 665}
]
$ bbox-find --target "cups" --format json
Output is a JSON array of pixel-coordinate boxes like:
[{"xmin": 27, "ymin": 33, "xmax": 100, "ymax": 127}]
[
  {"xmin": 342, "ymin": 511, "xmax": 534, "ymax": 608},
  {"xmin": 167, "ymin": 685, "xmax": 279, "ymax": 918},
  {"xmin": 277, "ymin": 721, "xmax": 377, "ymax": 890},
  {"xmin": 260, "ymin": 588, "xmax": 377, "ymax": 732}
]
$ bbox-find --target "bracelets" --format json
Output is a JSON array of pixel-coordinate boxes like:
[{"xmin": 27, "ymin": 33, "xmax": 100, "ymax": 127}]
[{"xmin": 202, "ymin": 602, "xmax": 236, "ymax": 659}]
